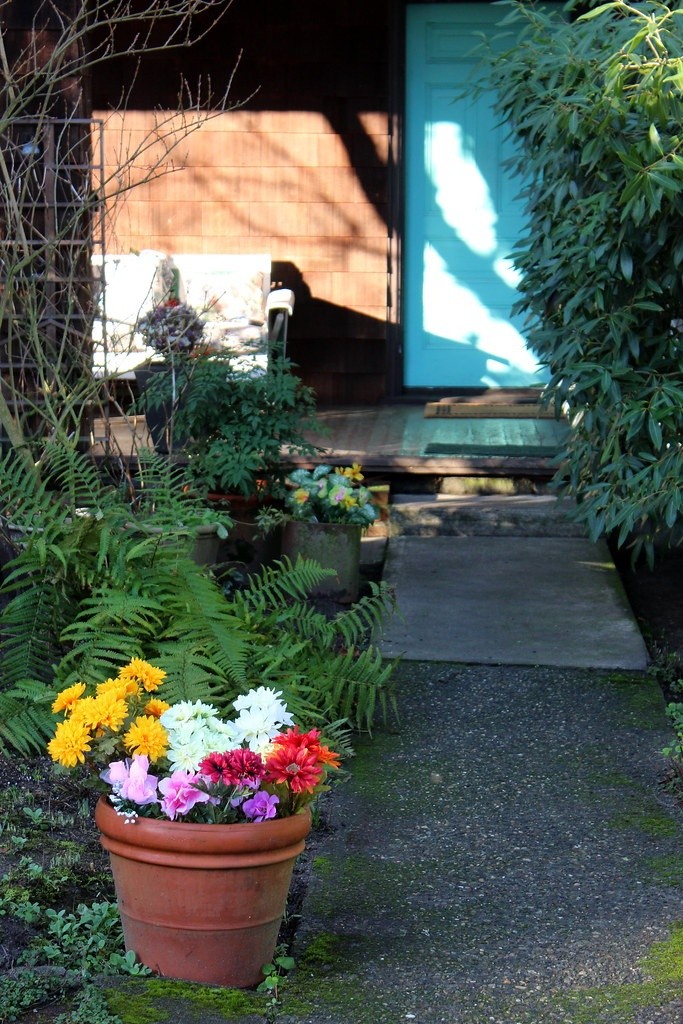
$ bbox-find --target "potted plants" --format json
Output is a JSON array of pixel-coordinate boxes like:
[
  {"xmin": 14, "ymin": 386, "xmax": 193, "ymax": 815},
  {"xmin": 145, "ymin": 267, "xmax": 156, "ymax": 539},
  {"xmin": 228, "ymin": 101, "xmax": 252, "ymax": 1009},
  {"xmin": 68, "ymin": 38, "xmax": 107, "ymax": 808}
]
[
  {"xmin": 126, "ymin": 337, "xmax": 336, "ymax": 541},
  {"xmin": 1, "ymin": 0, "xmax": 263, "ymax": 574}
]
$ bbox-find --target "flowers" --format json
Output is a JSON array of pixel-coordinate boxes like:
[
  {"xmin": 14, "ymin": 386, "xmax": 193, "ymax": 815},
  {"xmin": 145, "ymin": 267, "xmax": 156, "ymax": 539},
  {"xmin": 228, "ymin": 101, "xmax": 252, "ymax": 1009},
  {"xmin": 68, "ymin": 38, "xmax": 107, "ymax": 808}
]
[
  {"xmin": 136, "ymin": 303, "xmax": 204, "ymax": 368},
  {"xmin": 47, "ymin": 656, "xmax": 342, "ymax": 824},
  {"xmin": 285, "ymin": 461, "xmax": 379, "ymax": 528}
]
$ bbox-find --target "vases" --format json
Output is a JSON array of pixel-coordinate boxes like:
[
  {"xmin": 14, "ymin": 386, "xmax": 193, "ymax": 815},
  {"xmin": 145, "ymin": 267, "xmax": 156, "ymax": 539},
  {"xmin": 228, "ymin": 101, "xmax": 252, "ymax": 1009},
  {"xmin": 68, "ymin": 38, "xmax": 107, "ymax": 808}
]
[
  {"xmin": 133, "ymin": 361, "xmax": 228, "ymax": 454},
  {"xmin": 368, "ymin": 485, "xmax": 390, "ymax": 504},
  {"xmin": 95, "ymin": 794, "xmax": 312, "ymax": 988},
  {"xmin": 361, "ymin": 535, "xmax": 389, "ymax": 601},
  {"xmin": 119, "ymin": 506, "xmax": 222, "ymax": 576},
  {"xmin": 281, "ymin": 520, "xmax": 362, "ymax": 604}
]
